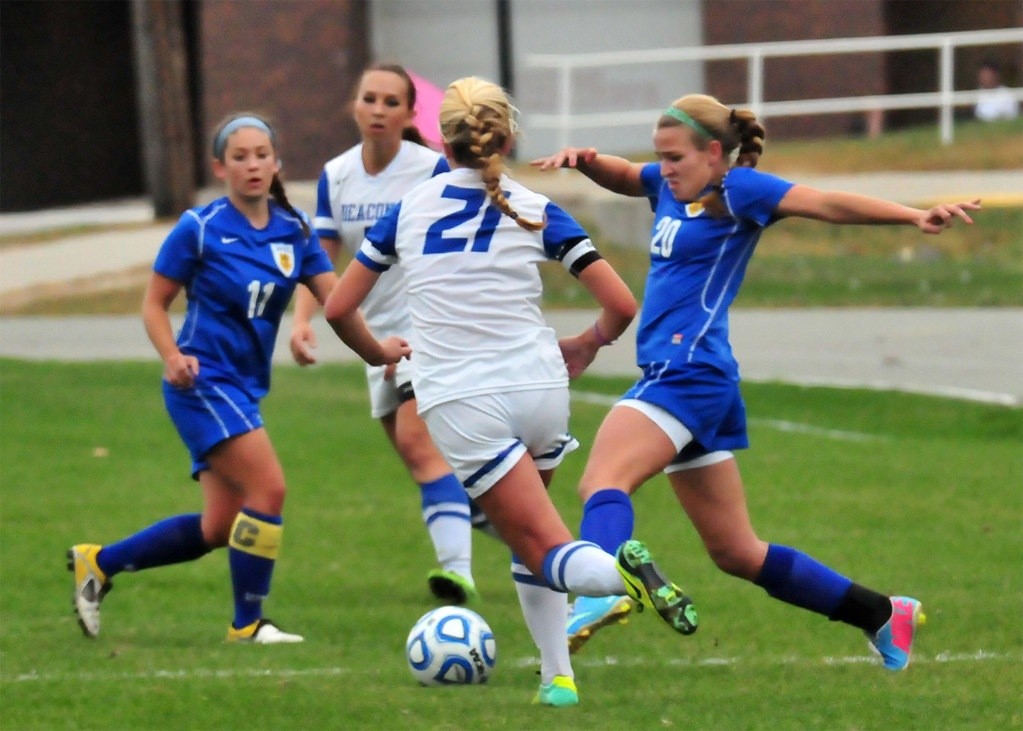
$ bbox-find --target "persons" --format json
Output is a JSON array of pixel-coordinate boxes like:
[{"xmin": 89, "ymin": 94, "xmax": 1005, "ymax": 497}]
[
  {"xmin": 321, "ymin": 75, "xmax": 700, "ymax": 705},
  {"xmin": 973, "ymin": 58, "xmax": 1023, "ymax": 121},
  {"xmin": 62, "ymin": 110, "xmax": 345, "ymax": 645},
  {"xmin": 527, "ymin": 93, "xmax": 981, "ymax": 675},
  {"xmin": 287, "ymin": 61, "xmax": 515, "ymax": 607}
]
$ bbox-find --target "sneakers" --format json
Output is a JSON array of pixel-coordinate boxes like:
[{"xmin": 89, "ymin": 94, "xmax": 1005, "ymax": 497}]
[
  {"xmin": 66, "ymin": 542, "xmax": 110, "ymax": 643},
  {"xmin": 613, "ymin": 536, "xmax": 700, "ymax": 636},
  {"xmin": 425, "ymin": 567, "xmax": 480, "ymax": 608},
  {"xmin": 226, "ymin": 618, "xmax": 309, "ymax": 645},
  {"xmin": 865, "ymin": 595, "xmax": 926, "ymax": 672},
  {"xmin": 527, "ymin": 675, "xmax": 580, "ymax": 710},
  {"xmin": 566, "ymin": 595, "xmax": 633, "ymax": 658}
]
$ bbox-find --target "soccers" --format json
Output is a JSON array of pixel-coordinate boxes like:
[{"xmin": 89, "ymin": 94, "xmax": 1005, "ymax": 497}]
[{"xmin": 405, "ymin": 606, "xmax": 497, "ymax": 689}]
